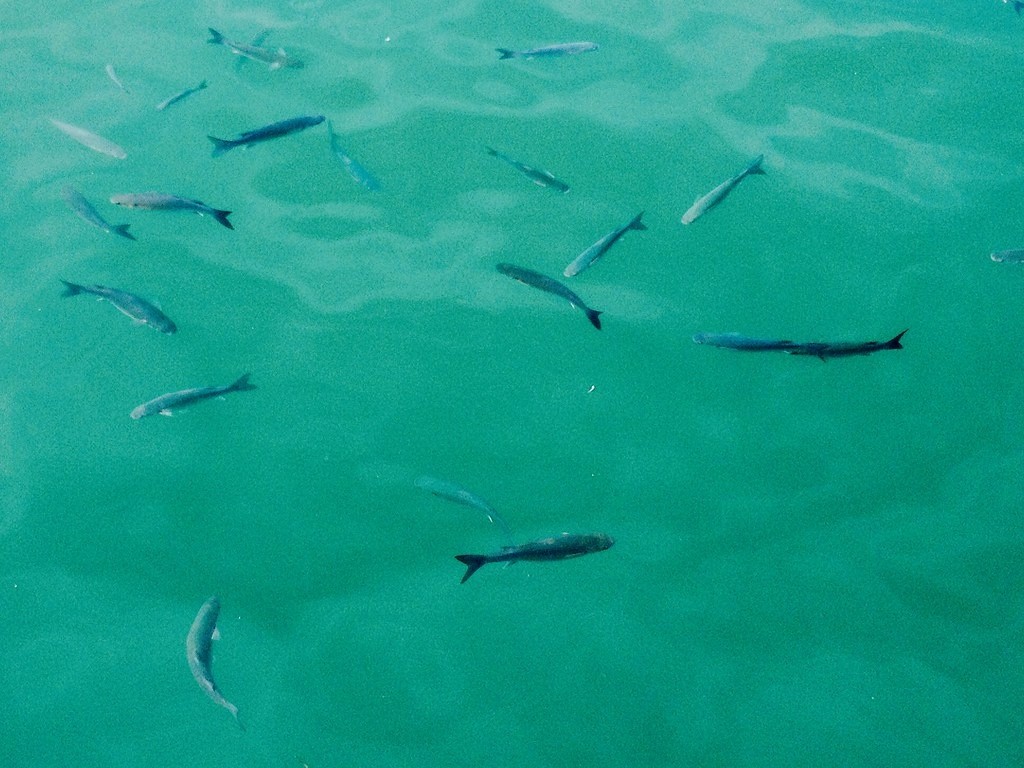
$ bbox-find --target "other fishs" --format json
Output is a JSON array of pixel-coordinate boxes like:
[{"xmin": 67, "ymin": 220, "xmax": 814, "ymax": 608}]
[
  {"xmin": 691, "ymin": 333, "xmax": 801, "ymax": 353},
  {"xmin": 155, "ymin": 79, "xmax": 209, "ymax": 113},
  {"xmin": 784, "ymin": 328, "xmax": 908, "ymax": 363},
  {"xmin": 483, "ymin": 145, "xmax": 570, "ymax": 196},
  {"xmin": 563, "ymin": 210, "xmax": 650, "ymax": 278},
  {"xmin": 110, "ymin": 192, "xmax": 235, "ymax": 232},
  {"xmin": 454, "ymin": 532, "xmax": 617, "ymax": 585},
  {"xmin": 185, "ymin": 596, "xmax": 249, "ymax": 732},
  {"xmin": 493, "ymin": 42, "xmax": 600, "ymax": 63},
  {"xmin": 130, "ymin": 371, "xmax": 258, "ymax": 421},
  {"xmin": 205, "ymin": 115, "xmax": 324, "ymax": 158},
  {"xmin": 105, "ymin": 63, "xmax": 130, "ymax": 95},
  {"xmin": 680, "ymin": 154, "xmax": 767, "ymax": 225},
  {"xmin": 49, "ymin": 118, "xmax": 127, "ymax": 160},
  {"xmin": 57, "ymin": 277, "xmax": 177, "ymax": 336},
  {"xmin": 206, "ymin": 25, "xmax": 305, "ymax": 73},
  {"xmin": 991, "ymin": 249, "xmax": 1024, "ymax": 264},
  {"xmin": 494, "ymin": 263, "xmax": 604, "ymax": 332},
  {"xmin": 328, "ymin": 120, "xmax": 384, "ymax": 198},
  {"xmin": 59, "ymin": 186, "xmax": 135, "ymax": 241},
  {"xmin": 412, "ymin": 476, "xmax": 517, "ymax": 547}
]
